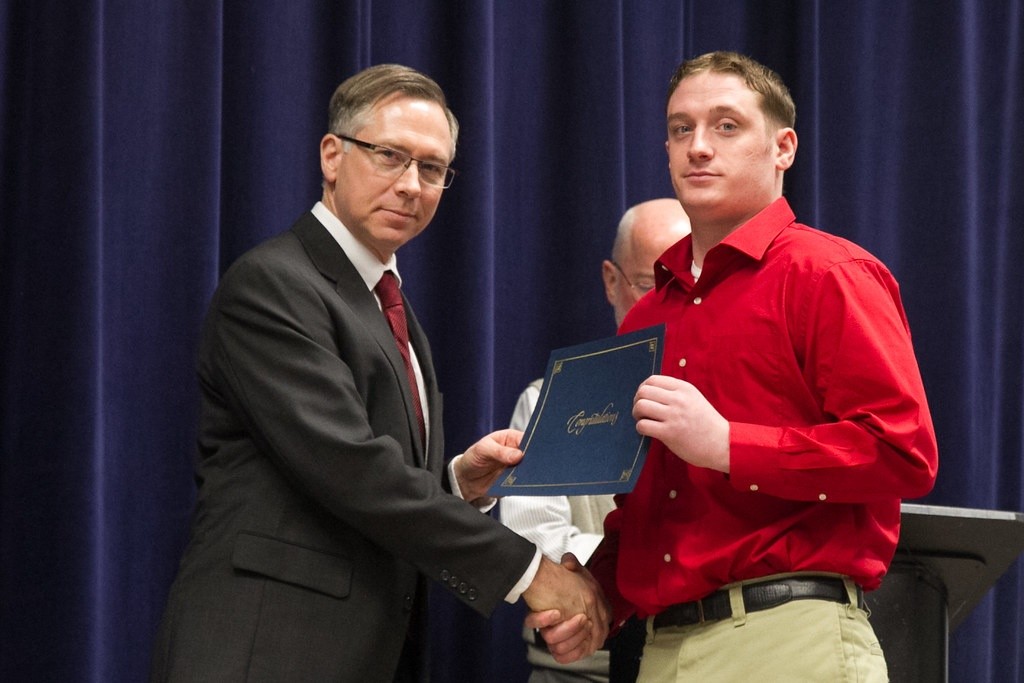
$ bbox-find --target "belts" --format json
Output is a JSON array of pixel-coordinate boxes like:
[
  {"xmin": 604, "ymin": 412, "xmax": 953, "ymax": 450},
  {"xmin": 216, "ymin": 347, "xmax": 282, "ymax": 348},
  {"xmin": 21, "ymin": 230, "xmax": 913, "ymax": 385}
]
[{"xmin": 660, "ymin": 577, "xmax": 866, "ymax": 629}]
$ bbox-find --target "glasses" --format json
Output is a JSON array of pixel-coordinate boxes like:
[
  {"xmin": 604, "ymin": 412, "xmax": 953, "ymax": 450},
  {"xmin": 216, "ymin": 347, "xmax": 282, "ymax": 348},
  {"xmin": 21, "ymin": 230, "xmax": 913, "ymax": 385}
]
[
  {"xmin": 338, "ymin": 133, "xmax": 455, "ymax": 189},
  {"xmin": 611, "ymin": 260, "xmax": 656, "ymax": 302}
]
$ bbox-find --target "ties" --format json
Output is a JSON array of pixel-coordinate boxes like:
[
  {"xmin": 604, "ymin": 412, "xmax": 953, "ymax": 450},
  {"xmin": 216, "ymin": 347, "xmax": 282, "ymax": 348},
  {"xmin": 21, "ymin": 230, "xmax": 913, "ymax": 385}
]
[{"xmin": 376, "ymin": 271, "xmax": 427, "ymax": 454}]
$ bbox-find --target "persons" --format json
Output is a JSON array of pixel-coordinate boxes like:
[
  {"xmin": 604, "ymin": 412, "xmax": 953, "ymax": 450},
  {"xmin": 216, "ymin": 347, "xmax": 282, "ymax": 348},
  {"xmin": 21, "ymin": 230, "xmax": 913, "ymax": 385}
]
[
  {"xmin": 495, "ymin": 48, "xmax": 942, "ymax": 683},
  {"xmin": 148, "ymin": 61, "xmax": 614, "ymax": 682}
]
